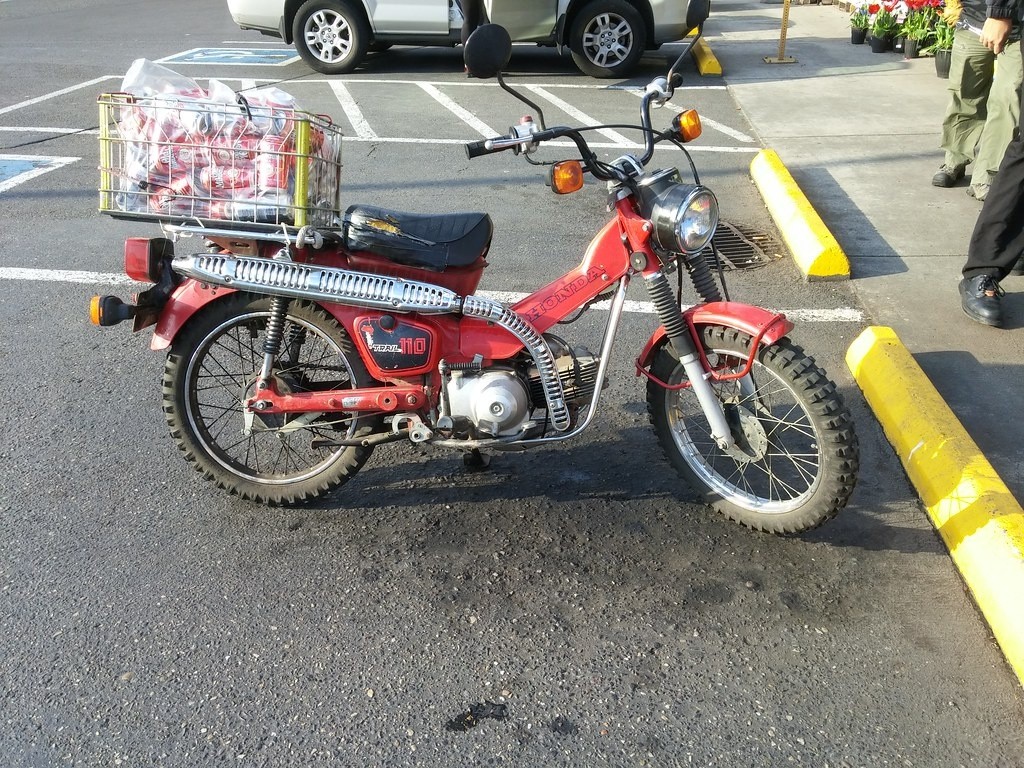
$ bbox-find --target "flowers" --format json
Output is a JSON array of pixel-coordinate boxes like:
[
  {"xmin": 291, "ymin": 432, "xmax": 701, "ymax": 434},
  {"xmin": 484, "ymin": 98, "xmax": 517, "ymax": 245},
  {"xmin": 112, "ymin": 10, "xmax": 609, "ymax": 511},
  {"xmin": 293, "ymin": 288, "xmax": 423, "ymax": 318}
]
[{"xmin": 849, "ymin": 0, "xmax": 963, "ymax": 54}]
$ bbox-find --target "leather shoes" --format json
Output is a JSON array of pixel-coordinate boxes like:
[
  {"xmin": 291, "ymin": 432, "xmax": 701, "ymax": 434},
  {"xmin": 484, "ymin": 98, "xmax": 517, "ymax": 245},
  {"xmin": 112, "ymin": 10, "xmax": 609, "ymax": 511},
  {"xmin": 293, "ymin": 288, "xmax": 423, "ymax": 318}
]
[
  {"xmin": 1008, "ymin": 250, "xmax": 1024, "ymax": 276},
  {"xmin": 958, "ymin": 275, "xmax": 1007, "ymax": 328}
]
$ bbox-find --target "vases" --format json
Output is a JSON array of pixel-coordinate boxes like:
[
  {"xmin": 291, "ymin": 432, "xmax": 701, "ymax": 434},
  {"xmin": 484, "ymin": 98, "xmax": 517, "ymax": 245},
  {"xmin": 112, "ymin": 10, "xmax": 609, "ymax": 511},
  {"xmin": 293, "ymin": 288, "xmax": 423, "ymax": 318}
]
[
  {"xmin": 926, "ymin": 36, "xmax": 936, "ymax": 47},
  {"xmin": 935, "ymin": 49, "xmax": 952, "ymax": 79},
  {"xmin": 871, "ymin": 34, "xmax": 889, "ymax": 53},
  {"xmin": 866, "ymin": 29, "xmax": 874, "ymax": 41},
  {"xmin": 887, "ymin": 33, "xmax": 896, "ymax": 50},
  {"xmin": 892, "ymin": 36, "xmax": 906, "ymax": 53},
  {"xmin": 904, "ymin": 39, "xmax": 919, "ymax": 58},
  {"xmin": 851, "ymin": 27, "xmax": 867, "ymax": 44}
]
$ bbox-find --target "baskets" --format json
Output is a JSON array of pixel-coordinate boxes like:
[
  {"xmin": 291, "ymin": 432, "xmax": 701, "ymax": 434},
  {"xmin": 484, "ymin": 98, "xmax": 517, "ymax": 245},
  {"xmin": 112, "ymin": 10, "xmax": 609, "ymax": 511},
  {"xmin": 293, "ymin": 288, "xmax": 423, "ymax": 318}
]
[{"xmin": 94, "ymin": 91, "xmax": 344, "ymax": 230}]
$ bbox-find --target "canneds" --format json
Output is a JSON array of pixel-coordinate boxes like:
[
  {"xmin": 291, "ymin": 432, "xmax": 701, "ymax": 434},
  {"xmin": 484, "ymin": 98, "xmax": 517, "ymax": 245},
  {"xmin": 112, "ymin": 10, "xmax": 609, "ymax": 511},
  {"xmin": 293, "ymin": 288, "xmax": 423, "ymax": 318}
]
[{"xmin": 121, "ymin": 88, "xmax": 324, "ymax": 221}]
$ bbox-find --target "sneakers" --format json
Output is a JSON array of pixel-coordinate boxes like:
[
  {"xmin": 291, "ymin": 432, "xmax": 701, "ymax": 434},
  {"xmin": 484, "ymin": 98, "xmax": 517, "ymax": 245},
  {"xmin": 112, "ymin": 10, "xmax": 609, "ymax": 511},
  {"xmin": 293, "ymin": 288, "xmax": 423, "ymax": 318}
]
[
  {"xmin": 966, "ymin": 182, "xmax": 990, "ymax": 202},
  {"xmin": 932, "ymin": 164, "xmax": 966, "ymax": 188}
]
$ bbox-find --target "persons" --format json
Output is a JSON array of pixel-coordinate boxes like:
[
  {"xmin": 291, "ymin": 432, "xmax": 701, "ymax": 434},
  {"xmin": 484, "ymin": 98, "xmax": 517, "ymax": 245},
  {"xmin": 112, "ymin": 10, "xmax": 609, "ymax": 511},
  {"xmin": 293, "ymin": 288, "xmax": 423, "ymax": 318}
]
[
  {"xmin": 931, "ymin": 0, "xmax": 1024, "ymax": 201},
  {"xmin": 957, "ymin": 0, "xmax": 1024, "ymax": 327}
]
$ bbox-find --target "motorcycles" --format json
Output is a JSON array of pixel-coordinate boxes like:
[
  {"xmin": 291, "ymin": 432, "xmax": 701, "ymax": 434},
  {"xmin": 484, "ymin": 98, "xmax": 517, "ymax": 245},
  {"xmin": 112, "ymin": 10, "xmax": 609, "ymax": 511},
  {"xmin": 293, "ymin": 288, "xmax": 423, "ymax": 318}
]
[{"xmin": 81, "ymin": 0, "xmax": 860, "ymax": 535}]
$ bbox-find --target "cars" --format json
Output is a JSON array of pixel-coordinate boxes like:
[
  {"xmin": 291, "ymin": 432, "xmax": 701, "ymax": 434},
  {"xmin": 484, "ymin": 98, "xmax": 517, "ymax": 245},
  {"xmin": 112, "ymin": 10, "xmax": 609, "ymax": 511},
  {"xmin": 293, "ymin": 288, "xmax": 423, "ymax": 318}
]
[{"xmin": 224, "ymin": 0, "xmax": 692, "ymax": 79}]
